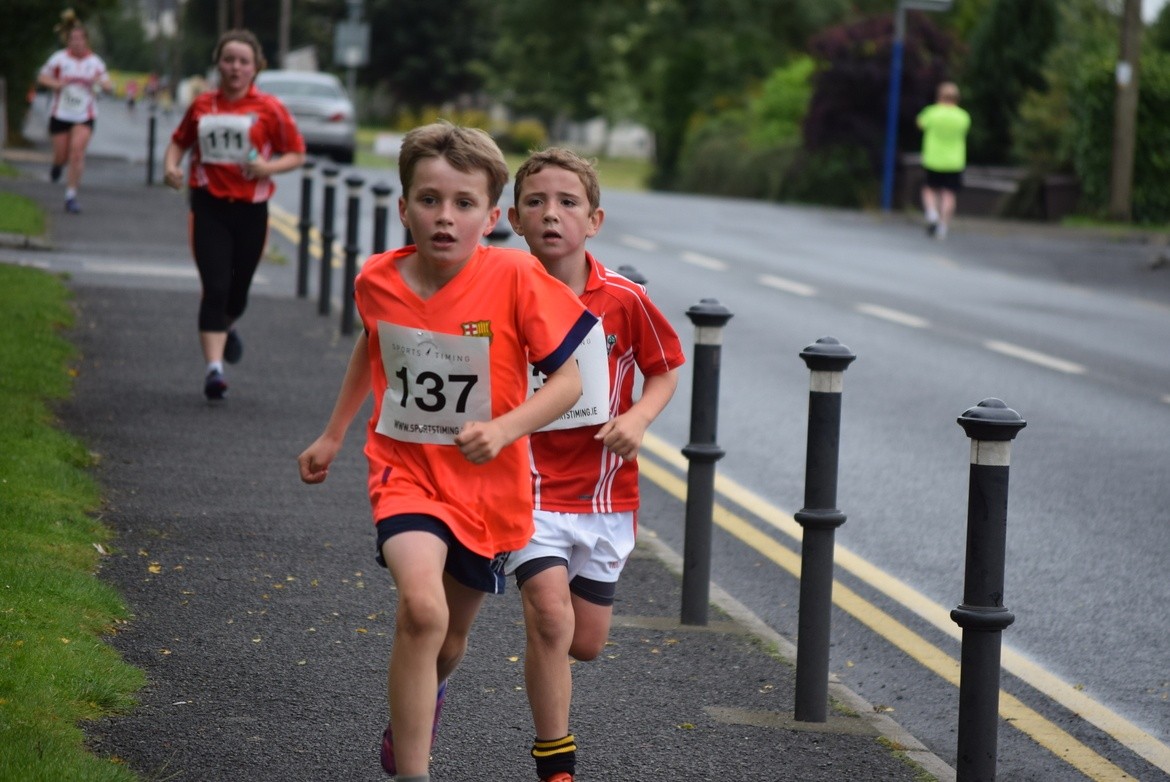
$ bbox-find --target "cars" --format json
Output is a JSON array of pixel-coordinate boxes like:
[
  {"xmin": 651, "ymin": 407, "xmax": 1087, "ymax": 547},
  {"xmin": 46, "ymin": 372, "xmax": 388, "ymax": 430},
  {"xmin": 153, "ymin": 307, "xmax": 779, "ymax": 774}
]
[{"xmin": 250, "ymin": 69, "xmax": 358, "ymax": 165}]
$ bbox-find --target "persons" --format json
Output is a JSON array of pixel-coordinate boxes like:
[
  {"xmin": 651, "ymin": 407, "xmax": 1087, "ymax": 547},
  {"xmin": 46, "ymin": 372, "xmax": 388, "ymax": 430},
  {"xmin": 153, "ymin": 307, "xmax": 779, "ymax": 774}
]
[
  {"xmin": 917, "ymin": 82, "xmax": 970, "ymax": 240},
  {"xmin": 27, "ymin": 71, "xmax": 206, "ymax": 123},
  {"xmin": 501, "ymin": 147, "xmax": 688, "ymax": 782},
  {"xmin": 163, "ymin": 31, "xmax": 306, "ymax": 400},
  {"xmin": 298, "ymin": 121, "xmax": 598, "ymax": 782},
  {"xmin": 37, "ymin": 10, "xmax": 113, "ymax": 218}
]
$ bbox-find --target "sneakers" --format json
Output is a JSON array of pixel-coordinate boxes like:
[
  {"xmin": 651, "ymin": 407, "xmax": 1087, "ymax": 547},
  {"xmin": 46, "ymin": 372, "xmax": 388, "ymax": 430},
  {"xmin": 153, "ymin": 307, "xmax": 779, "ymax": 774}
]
[
  {"xmin": 205, "ymin": 368, "xmax": 227, "ymax": 400},
  {"xmin": 381, "ymin": 676, "xmax": 448, "ymax": 776},
  {"xmin": 223, "ymin": 328, "xmax": 242, "ymax": 364}
]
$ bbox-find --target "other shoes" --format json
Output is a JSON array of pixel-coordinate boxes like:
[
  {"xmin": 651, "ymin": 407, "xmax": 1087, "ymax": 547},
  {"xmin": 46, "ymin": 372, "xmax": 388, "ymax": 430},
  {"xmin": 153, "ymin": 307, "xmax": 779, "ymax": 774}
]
[
  {"xmin": 925, "ymin": 213, "xmax": 939, "ymax": 236},
  {"xmin": 50, "ymin": 165, "xmax": 62, "ymax": 182},
  {"xmin": 936, "ymin": 225, "xmax": 946, "ymax": 241},
  {"xmin": 66, "ymin": 199, "xmax": 80, "ymax": 214},
  {"xmin": 541, "ymin": 771, "xmax": 575, "ymax": 782}
]
